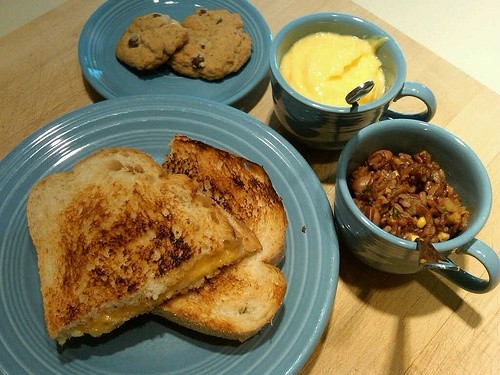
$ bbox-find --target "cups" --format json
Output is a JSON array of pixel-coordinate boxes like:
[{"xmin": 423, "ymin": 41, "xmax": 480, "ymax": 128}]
[
  {"xmin": 271, "ymin": 11, "xmax": 436, "ymax": 152},
  {"xmin": 333, "ymin": 118, "xmax": 500, "ymax": 295}
]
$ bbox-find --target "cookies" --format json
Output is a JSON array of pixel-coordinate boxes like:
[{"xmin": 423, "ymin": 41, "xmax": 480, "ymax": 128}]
[
  {"xmin": 171, "ymin": 8, "xmax": 253, "ymax": 82},
  {"xmin": 114, "ymin": 12, "xmax": 189, "ymax": 70}
]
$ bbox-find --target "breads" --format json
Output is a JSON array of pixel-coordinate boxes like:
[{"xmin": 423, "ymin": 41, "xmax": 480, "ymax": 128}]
[
  {"xmin": 27, "ymin": 148, "xmax": 264, "ymax": 347},
  {"xmin": 151, "ymin": 134, "xmax": 289, "ymax": 343}
]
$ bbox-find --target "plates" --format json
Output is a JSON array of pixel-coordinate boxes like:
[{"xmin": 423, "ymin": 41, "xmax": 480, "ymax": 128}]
[
  {"xmin": 78, "ymin": 0, "xmax": 271, "ymax": 104},
  {"xmin": 0, "ymin": 97, "xmax": 342, "ymax": 374}
]
